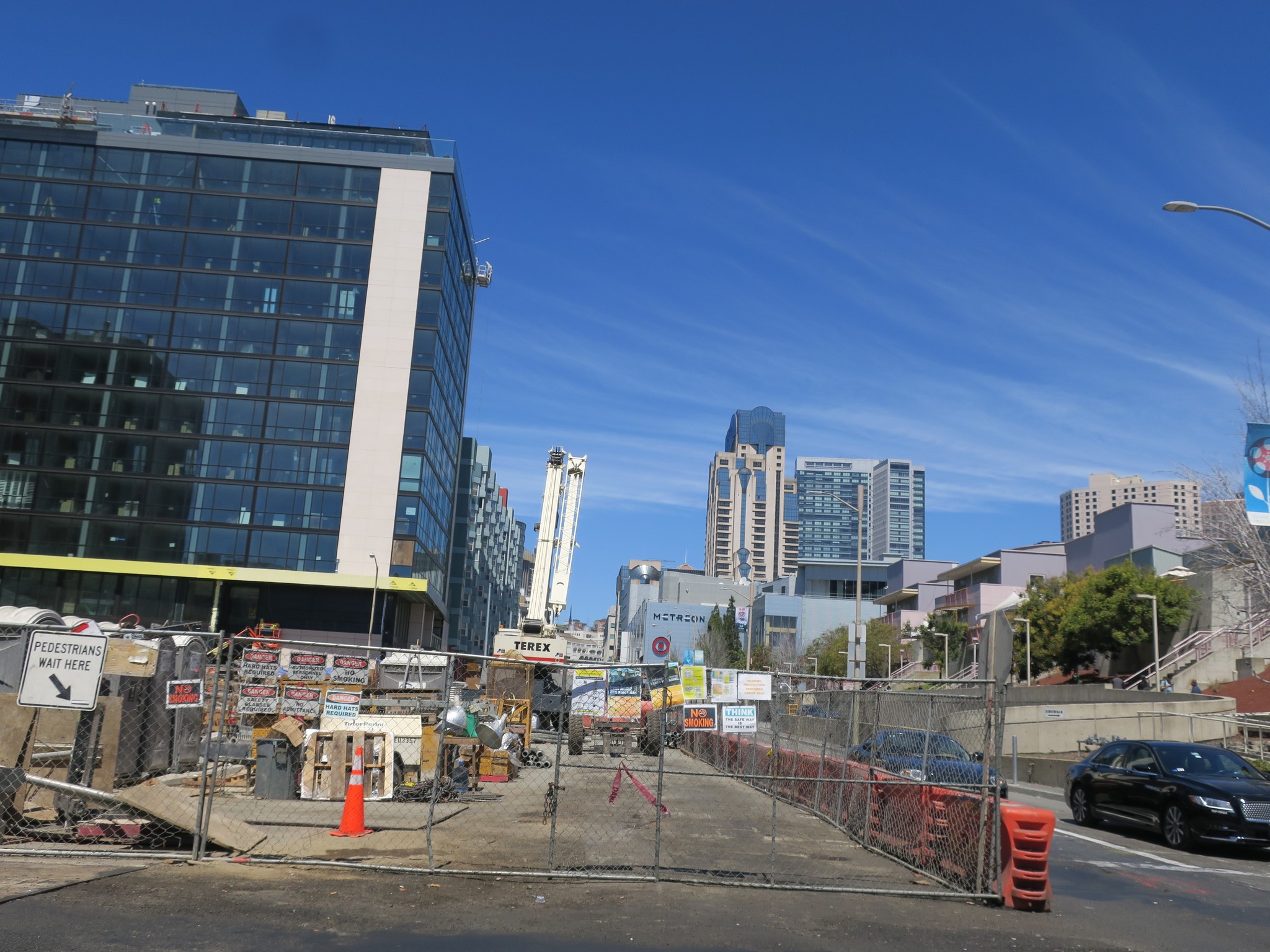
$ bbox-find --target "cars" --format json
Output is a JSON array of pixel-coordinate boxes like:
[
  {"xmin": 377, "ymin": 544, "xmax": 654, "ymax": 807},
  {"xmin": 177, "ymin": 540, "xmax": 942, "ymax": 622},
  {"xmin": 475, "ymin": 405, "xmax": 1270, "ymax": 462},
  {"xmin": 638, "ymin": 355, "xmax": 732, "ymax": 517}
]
[
  {"xmin": 1062, "ymin": 739, "xmax": 1270, "ymax": 857},
  {"xmin": 847, "ymin": 728, "xmax": 1007, "ymax": 801},
  {"xmin": 795, "ymin": 705, "xmax": 843, "ymax": 720}
]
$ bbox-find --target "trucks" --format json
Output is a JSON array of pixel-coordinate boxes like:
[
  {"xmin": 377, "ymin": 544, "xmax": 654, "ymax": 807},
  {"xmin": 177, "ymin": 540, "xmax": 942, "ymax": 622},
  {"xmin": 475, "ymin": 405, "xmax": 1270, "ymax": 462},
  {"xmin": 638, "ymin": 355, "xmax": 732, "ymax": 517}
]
[{"xmin": 377, "ymin": 648, "xmax": 450, "ymax": 723}]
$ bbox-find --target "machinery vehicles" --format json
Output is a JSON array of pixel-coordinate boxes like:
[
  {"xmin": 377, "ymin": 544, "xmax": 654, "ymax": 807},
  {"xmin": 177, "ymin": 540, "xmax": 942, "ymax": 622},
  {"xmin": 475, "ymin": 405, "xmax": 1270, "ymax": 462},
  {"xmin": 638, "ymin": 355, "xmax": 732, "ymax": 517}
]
[
  {"xmin": 568, "ymin": 675, "xmax": 662, "ymax": 757},
  {"xmin": 483, "ymin": 444, "xmax": 588, "ymax": 727}
]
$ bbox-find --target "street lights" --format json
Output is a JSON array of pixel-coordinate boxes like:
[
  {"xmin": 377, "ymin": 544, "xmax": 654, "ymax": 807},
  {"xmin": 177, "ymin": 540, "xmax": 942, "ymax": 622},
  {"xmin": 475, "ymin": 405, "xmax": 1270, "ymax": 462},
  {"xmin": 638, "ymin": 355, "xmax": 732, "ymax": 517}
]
[
  {"xmin": 651, "ymin": 624, "xmax": 672, "ymax": 662},
  {"xmin": 718, "ymin": 584, "xmax": 755, "ymax": 671},
  {"xmin": 674, "ymin": 606, "xmax": 697, "ymax": 666},
  {"xmin": 366, "ymin": 552, "xmax": 379, "ymax": 660},
  {"xmin": 973, "ymin": 642, "xmax": 980, "ymax": 681},
  {"xmin": 838, "ymin": 651, "xmax": 849, "ymax": 677},
  {"xmin": 899, "ymin": 649, "xmax": 904, "ymax": 678},
  {"xmin": 934, "ymin": 632, "xmax": 948, "ymax": 689},
  {"xmin": 1014, "ymin": 617, "xmax": 1031, "ymax": 687},
  {"xmin": 762, "ymin": 666, "xmax": 771, "ymax": 672},
  {"xmin": 807, "ymin": 657, "xmax": 817, "ymax": 690},
  {"xmin": 783, "ymin": 662, "xmax": 792, "ymax": 691},
  {"xmin": 877, "ymin": 644, "xmax": 891, "ymax": 688},
  {"xmin": 1131, "ymin": 593, "xmax": 1161, "ymax": 690},
  {"xmin": 807, "ymin": 483, "xmax": 863, "ymax": 746}
]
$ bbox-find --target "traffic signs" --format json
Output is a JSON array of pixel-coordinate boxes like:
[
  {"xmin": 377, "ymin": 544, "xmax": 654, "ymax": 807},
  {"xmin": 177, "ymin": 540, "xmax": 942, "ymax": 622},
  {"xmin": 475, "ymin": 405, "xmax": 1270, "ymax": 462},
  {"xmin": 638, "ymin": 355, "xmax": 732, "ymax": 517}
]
[{"xmin": 15, "ymin": 630, "xmax": 107, "ymax": 711}]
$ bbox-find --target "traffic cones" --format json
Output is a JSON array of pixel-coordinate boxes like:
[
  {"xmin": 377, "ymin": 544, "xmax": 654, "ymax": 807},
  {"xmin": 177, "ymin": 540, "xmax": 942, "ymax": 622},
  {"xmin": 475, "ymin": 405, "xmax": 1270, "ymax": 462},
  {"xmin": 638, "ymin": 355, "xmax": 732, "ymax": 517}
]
[{"xmin": 329, "ymin": 747, "xmax": 376, "ymax": 837}]
